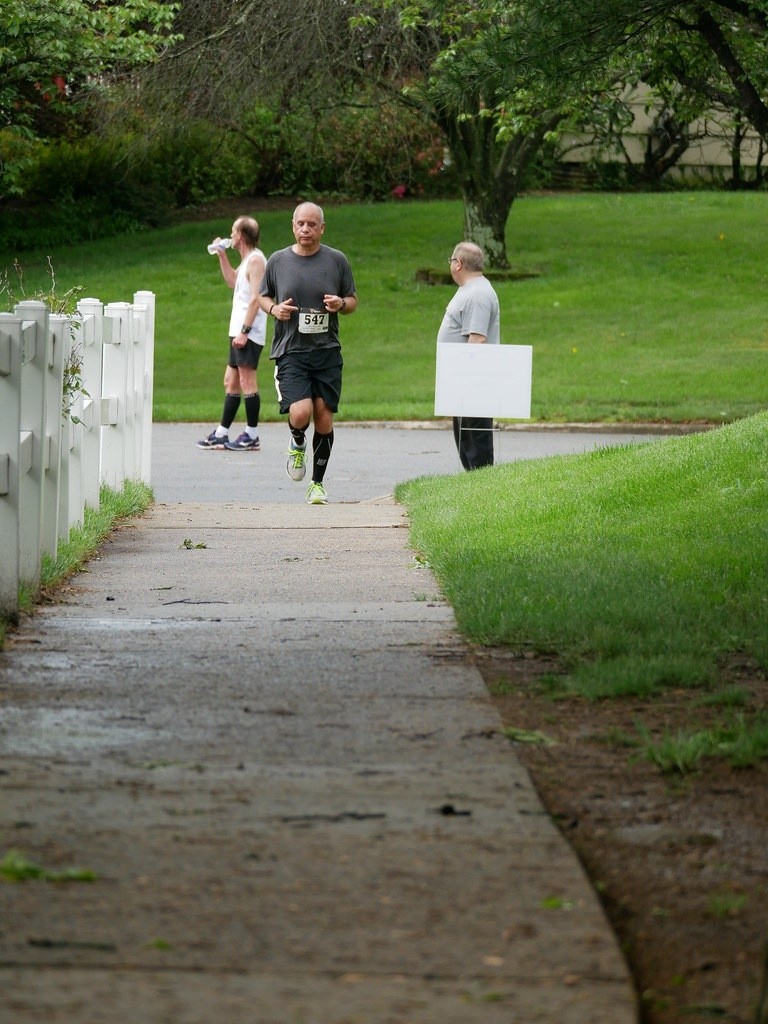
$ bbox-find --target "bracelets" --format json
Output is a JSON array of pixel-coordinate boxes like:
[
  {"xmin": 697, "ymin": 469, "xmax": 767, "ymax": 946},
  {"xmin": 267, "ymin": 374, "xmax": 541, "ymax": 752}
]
[
  {"xmin": 270, "ymin": 304, "xmax": 275, "ymax": 317},
  {"xmin": 338, "ymin": 298, "xmax": 347, "ymax": 312}
]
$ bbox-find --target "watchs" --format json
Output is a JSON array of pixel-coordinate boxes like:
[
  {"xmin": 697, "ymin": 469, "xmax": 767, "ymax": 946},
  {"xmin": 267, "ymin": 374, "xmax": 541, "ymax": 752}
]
[{"xmin": 240, "ymin": 325, "xmax": 252, "ymax": 334}]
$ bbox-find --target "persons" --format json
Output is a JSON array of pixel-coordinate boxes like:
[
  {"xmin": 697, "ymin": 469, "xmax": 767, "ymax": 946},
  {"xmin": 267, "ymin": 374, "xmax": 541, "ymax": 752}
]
[
  {"xmin": 435, "ymin": 240, "xmax": 501, "ymax": 473},
  {"xmin": 192, "ymin": 213, "xmax": 270, "ymax": 452},
  {"xmin": 255, "ymin": 200, "xmax": 359, "ymax": 505}
]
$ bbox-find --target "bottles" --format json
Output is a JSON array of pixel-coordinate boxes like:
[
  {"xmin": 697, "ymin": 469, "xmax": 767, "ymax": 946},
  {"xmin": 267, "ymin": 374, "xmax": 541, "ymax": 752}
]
[{"xmin": 207, "ymin": 239, "xmax": 234, "ymax": 255}]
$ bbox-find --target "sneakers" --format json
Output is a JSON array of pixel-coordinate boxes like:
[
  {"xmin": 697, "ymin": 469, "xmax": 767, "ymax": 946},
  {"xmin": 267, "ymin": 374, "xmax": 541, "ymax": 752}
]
[
  {"xmin": 304, "ymin": 480, "xmax": 328, "ymax": 505},
  {"xmin": 224, "ymin": 432, "xmax": 260, "ymax": 450},
  {"xmin": 286, "ymin": 435, "xmax": 307, "ymax": 482},
  {"xmin": 196, "ymin": 430, "xmax": 229, "ymax": 449}
]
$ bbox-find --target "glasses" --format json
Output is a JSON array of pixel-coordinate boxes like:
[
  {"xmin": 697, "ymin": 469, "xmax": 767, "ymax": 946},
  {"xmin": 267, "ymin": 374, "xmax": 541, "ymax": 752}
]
[{"xmin": 448, "ymin": 258, "xmax": 457, "ymax": 264}]
